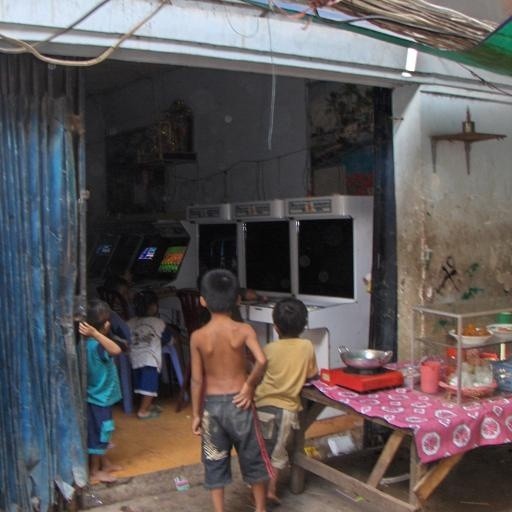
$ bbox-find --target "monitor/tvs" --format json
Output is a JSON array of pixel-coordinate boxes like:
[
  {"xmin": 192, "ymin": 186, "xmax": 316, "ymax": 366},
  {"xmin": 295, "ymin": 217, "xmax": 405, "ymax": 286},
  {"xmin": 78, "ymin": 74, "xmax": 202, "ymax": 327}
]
[
  {"xmin": 197, "ymin": 222, "xmax": 238, "ymax": 285},
  {"xmin": 244, "ymin": 220, "xmax": 290, "ymax": 294},
  {"xmin": 137, "ymin": 246, "xmax": 156, "ymax": 260},
  {"xmin": 94, "ymin": 243, "xmax": 115, "ymax": 259},
  {"xmin": 158, "ymin": 246, "xmax": 185, "ymax": 274},
  {"xmin": 299, "ymin": 219, "xmax": 354, "ymax": 298}
]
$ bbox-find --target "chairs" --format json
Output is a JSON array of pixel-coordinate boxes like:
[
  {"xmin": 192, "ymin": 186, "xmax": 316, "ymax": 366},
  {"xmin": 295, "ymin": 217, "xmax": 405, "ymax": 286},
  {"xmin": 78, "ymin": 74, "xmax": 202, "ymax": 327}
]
[{"xmin": 96, "ymin": 286, "xmax": 189, "ymax": 416}]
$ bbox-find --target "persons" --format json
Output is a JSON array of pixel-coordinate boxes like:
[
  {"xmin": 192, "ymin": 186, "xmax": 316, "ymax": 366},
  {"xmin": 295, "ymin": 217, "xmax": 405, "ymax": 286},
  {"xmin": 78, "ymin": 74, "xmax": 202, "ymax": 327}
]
[
  {"xmin": 252, "ymin": 298, "xmax": 319, "ymax": 505},
  {"xmin": 333, "ymin": 118, "xmax": 377, "ymax": 195},
  {"xmin": 188, "ymin": 267, "xmax": 276, "ymax": 511},
  {"xmin": 77, "ymin": 249, "xmax": 189, "ymax": 483}
]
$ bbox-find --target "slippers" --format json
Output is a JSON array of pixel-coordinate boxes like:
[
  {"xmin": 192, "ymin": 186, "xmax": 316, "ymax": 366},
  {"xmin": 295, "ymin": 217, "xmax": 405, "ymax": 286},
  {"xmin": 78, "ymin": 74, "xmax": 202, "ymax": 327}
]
[
  {"xmin": 150, "ymin": 405, "xmax": 163, "ymax": 411},
  {"xmin": 138, "ymin": 411, "xmax": 159, "ymax": 419}
]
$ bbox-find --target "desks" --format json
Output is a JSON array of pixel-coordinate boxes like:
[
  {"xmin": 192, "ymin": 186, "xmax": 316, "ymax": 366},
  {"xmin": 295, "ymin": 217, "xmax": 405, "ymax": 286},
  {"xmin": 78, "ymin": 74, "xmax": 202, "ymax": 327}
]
[{"xmin": 286, "ymin": 355, "xmax": 512, "ymax": 512}]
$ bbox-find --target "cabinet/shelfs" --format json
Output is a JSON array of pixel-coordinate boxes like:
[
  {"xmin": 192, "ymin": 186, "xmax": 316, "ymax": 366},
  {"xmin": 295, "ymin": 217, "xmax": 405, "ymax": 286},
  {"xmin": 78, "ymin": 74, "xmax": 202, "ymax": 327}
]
[{"xmin": 411, "ymin": 297, "xmax": 512, "ymax": 404}]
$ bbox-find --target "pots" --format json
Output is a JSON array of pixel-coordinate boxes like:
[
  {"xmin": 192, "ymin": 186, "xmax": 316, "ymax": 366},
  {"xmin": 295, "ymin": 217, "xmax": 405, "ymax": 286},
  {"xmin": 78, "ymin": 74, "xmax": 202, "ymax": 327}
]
[{"xmin": 337, "ymin": 345, "xmax": 393, "ymax": 370}]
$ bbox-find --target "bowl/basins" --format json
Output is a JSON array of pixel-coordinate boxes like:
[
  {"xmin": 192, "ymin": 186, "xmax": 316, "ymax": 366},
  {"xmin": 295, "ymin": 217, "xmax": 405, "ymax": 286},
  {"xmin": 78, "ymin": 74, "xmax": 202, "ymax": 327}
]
[
  {"xmin": 486, "ymin": 323, "xmax": 512, "ymax": 341},
  {"xmin": 448, "ymin": 327, "xmax": 494, "ymax": 344}
]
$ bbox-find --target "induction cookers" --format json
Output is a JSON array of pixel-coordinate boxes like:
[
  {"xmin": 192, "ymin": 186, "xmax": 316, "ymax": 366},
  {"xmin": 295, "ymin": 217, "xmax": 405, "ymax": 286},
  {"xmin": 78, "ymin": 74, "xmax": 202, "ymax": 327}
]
[{"xmin": 319, "ymin": 367, "xmax": 404, "ymax": 394}]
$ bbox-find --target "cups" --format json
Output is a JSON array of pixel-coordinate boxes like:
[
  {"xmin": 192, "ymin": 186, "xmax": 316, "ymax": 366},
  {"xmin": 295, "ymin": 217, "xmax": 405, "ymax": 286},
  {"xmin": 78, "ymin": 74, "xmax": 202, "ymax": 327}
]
[
  {"xmin": 420, "ymin": 360, "xmax": 441, "ymax": 395},
  {"xmin": 495, "ymin": 312, "xmax": 512, "ymax": 324},
  {"xmin": 474, "ymin": 361, "xmax": 494, "ymax": 386}
]
